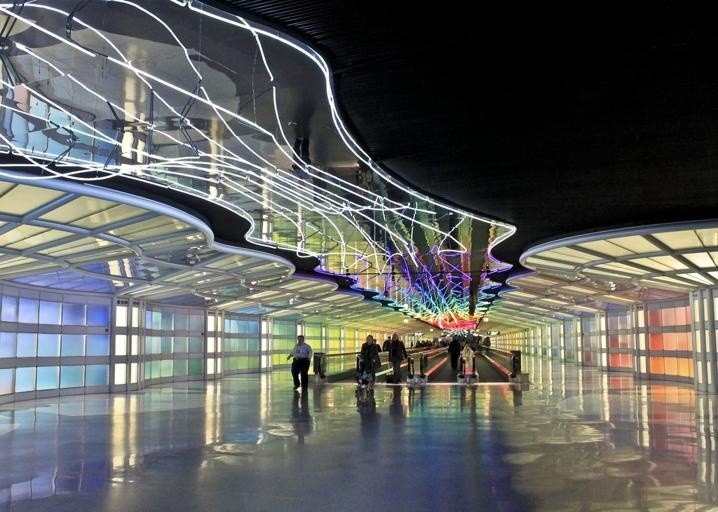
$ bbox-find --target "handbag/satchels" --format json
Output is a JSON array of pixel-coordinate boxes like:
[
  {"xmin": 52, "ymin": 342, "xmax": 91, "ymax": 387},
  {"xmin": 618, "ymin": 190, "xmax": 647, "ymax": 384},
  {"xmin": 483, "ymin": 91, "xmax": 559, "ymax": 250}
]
[{"xmin": 357, "ymin": 360, "xmax": 373, "ymax": 384}]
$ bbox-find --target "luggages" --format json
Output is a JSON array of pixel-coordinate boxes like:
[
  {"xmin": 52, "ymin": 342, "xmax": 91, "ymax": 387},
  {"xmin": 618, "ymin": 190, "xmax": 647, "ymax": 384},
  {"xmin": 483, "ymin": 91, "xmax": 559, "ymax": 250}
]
[
  {"xmin": 356, "ymin": 364, "xmax": 374, "ymax": 407},
  {"xmin": 385, "ymin": 361, "xmax": 394, "ymax": 383}
]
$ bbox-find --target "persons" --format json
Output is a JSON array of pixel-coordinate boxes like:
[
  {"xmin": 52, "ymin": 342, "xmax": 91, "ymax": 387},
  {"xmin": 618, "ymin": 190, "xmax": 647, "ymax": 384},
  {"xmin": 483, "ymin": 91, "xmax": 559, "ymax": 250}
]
[
  {"xmin": 359, "ymin": 330, "xmax": 479, "ymax": 388},
  {"xmin": 286, "ymin": 335, "xmax": 312, "ymax": 390}
]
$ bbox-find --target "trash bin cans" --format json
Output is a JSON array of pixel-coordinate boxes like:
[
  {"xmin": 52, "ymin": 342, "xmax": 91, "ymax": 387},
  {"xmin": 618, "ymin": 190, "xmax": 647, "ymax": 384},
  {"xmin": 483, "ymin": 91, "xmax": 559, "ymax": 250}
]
[
  {"xmin": 314, "ymin": 353, "xmax": 325, "ymax": 378},
  {"xmin": 511, "ymin": 351, "xmax": 521, "ymax": 377}
]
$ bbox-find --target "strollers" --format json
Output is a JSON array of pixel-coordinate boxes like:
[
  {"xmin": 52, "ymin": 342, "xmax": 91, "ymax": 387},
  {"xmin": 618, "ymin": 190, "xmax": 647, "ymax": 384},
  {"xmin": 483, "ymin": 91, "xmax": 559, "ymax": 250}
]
[{"xmin": 355, "ymin": 361, "xmax": 375, "ymax": 393}]
